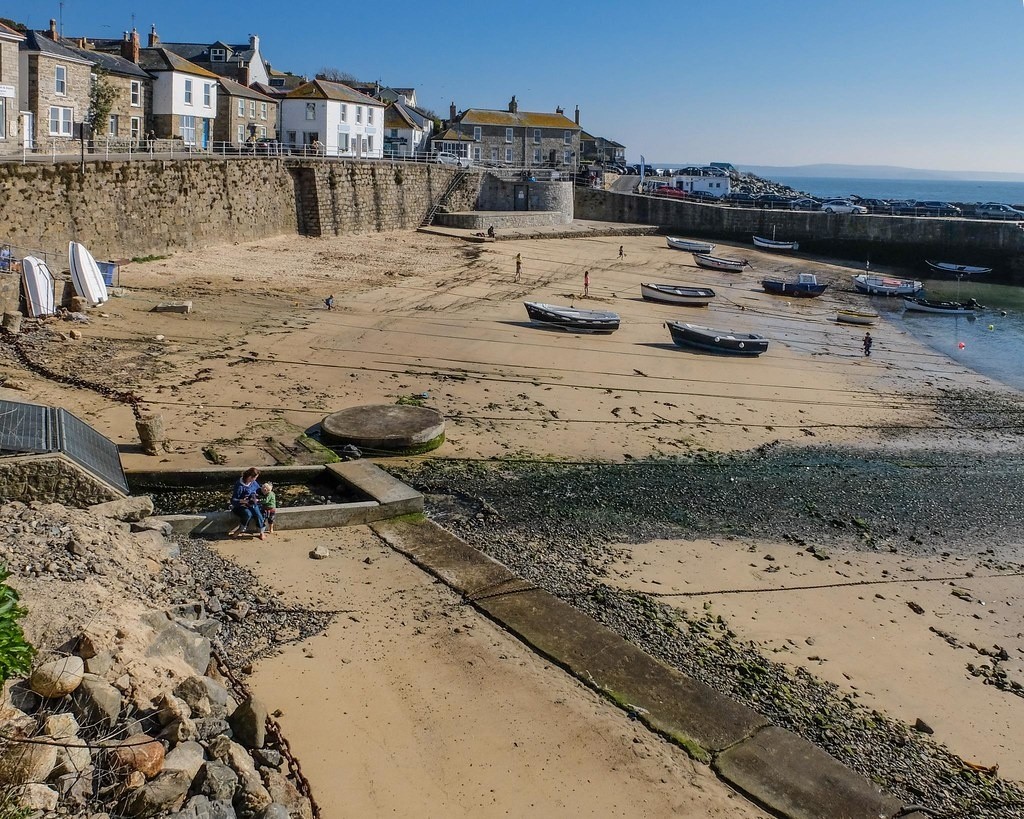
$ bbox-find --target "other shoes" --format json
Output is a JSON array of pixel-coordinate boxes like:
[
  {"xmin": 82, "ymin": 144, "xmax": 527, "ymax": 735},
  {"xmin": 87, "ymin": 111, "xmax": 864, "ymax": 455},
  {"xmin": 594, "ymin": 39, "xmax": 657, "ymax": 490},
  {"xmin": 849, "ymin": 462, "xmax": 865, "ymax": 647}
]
[
  {"xmin": 270, "ymin": 524, "xmax": 274, "ymax": 534},
  {"xmin": 232, "ymin": 531, "xmax": 241, "ymax": 539},
  {"xmin": 260, "ymin": 532, "xmax": 266, "ymax": 540}
]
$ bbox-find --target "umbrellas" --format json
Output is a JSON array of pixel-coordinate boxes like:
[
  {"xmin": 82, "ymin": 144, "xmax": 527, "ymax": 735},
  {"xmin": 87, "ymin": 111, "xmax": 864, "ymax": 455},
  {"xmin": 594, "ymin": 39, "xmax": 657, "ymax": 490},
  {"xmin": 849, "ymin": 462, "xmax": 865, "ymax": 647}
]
[{"xmin": 925, "ymin": 260, "xmax": 992, "ymax": 306}]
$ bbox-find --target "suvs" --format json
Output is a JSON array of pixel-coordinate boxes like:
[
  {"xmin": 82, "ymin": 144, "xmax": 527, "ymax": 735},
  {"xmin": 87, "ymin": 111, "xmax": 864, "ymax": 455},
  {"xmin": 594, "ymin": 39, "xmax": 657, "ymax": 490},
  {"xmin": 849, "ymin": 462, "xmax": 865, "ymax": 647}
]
[{"xmin": 431, "ymin": 151, "xmax": 463, "ymax": 167}]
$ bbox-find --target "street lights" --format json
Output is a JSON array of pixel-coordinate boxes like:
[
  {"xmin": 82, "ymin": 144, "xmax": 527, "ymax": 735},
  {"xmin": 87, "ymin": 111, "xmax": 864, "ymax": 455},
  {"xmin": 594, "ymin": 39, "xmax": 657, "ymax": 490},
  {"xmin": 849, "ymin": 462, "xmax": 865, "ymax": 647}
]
[{"xmin": 457, "ymin": 109, "xmax": 463, "ymax": 162}]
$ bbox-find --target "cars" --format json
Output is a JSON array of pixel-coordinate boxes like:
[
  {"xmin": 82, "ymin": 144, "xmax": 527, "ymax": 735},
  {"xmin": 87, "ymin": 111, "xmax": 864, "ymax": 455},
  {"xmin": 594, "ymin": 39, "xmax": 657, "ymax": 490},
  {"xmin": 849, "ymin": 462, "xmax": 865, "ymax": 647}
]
[
  {"xmin": 821, "ymin": 200, "xmax": 867, "ymax": 214},
  {"xmin": 608, "ymin": 166, "xmax": 730, "ymax": 177},
  {"xmin": 653, "ymin": 185, "xmax": 822, "ymax": 211},
  {"xmin": 858, "ymin": 197, "xmax": 1024, "ymax": 220},
  {"xmin": 256, "ymin": 138, "xmax": 292, "ymax": 156}
]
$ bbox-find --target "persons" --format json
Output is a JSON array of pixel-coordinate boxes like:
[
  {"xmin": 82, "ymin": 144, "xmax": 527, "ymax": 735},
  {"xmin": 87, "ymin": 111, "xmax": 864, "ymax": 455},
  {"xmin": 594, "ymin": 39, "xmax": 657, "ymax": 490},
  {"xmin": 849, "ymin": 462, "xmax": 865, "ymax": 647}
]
[
  {"xmin": 520, "ymin": 169, "xmax": 526, "ymax": 176},
  {"xmin": 471, "ymin": 226, "xmax": 495, "ymax": 237},
  {"xmin": 1, "ymin": 245, "xmax": 15, "ymax": 264},
  {"xmin": 259, "ymin": 483, "xmax": 276, "ymax": 532},
  {"xmin": 584, "ymin": 271, "xmax": 589, "ymax": 298},
  {"xmin": 248, "ymin": 132, "xmax": 257, "ymax": 152},
  {"xmin": 617, "ymin": 245, "xmax": 624, "ymax": 259},
  {"xmin": 863, "ymin": 332, "xmax": 872, "ymax": 356},
  {"xmin": 528, "ymin": 170, "xmax": 533, "ymax": 177},
  {"xmin": 515, "ymin": 252, "xmax": 522, "ymax": 281},
  {"xmin": 312, "ymin": 140, "xmax": 324, "ymax": 155},
  {"xmin": 147, "ymin": 130, "xmax": 157, "ymax": 153},
  {"xmin": 588, "ymin": 172, "xmax": 596, "ymax": 187},
  {"xmin": 231, "ymin": 467, "xmax": 266, "ymax": 540}
]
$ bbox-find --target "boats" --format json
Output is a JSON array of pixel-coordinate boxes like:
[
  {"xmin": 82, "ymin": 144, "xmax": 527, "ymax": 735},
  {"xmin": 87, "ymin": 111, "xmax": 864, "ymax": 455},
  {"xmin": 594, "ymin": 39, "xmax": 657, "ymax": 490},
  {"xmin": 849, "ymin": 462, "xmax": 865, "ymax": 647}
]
[
  {"xmin": 757, "ymin": 273, "xmax": 830, "ymax": 297},
  {"xmin": 21, "ymin": 255, "xmax": 57, "ymax": 317},
  {"xmin": 665, "ymin": 320, "xmax": 770, "ymax": 354},
  {"xmin": 666, "ymin": 236, "xmax": 717, "ymax": 254},
  {"xmin": 925, "ymin": 259, "xmax": 992, "ymax": 276},
  {"xmin": 850, "ymin": 274, "xmax": 923, "ymax": 295},
  {"xmin": 523, "ymin": 301, "xmax": 621, "ymax": 333},
  {"xmin": 692, "ymin": 251, "xmax": 754, "ymax": 272},
  {"xmin": 641, "ymin": 282, "xmax": 716, "ymax": 306},
  {"xmin": 753, "ymin": 235, "xmax": 799, "ymax": 250},
  {"xmin": 69, "ymin": 241, "xmax": 108, "ymax": 308},
  {"xmin": 836, "ymin": 309, "xmax": 879, "ymax": 324},
  {"xmin": 902, "ymin": 294, "xmax": 985, "ymax": 314}
]
